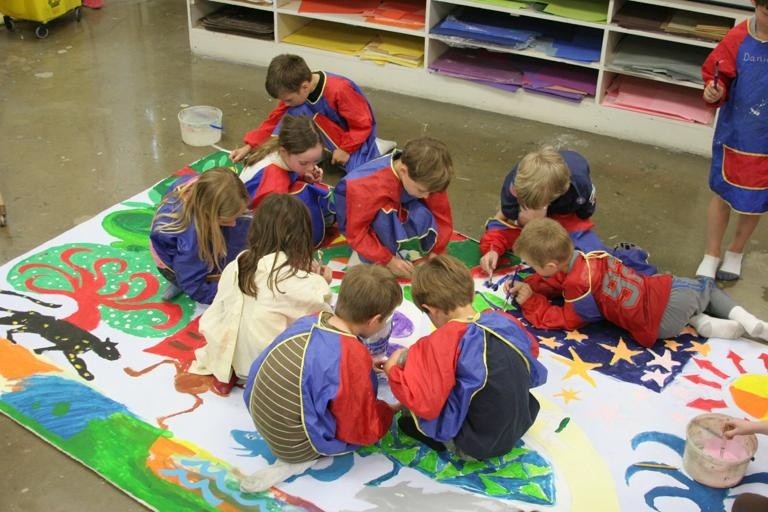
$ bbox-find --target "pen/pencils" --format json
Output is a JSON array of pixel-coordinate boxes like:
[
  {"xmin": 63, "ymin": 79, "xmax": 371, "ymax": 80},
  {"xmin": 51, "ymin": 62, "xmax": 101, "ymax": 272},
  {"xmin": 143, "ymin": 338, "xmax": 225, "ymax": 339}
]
[{"xmin": 634, "ymin": 463, "xmax": 680, "ymax": 470}]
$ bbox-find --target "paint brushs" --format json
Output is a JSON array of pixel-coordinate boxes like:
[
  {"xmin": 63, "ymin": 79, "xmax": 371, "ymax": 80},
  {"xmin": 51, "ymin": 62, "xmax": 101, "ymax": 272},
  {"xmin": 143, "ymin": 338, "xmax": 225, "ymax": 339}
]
[
  {"xmin": 715, "ymin": 61, "xmax": 719, "ymax": 89},
  {"xmin": 490, "ymin": 246, "xmax": 494, "ymax": 283},
  {"xmin": 504, "ymin": 270, "xmax": 518, "ymax": 308},
  {"xmin": 234, "ymin": 145, "xmax": 237, "ymax": 158},
  {"xmin": 395, "ymin": 248, "xmax": 403, "ymax": 258}
]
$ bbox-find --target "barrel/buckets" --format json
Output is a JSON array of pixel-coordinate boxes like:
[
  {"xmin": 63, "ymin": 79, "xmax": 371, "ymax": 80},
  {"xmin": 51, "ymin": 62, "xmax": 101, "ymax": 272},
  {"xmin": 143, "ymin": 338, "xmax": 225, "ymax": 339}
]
[
  {"xmin": 178, "ymin": 105, "xmax": 224, "ymax": 147},
  {"xmin": 682, "ymin": 412, "xmax": 758, "ymax": 489}
]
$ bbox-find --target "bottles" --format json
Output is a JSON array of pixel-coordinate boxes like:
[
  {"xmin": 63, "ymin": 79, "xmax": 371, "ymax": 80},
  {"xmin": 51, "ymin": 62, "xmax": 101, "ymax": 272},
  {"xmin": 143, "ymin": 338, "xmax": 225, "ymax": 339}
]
[{"xmin": 365, "ymin": 321, "xmax": 389, "ymax": 357}]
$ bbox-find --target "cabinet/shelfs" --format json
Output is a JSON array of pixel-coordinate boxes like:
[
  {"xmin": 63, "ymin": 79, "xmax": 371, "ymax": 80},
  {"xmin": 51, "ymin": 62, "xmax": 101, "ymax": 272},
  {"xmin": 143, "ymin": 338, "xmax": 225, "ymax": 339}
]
[{"xmin": 186, "ymin": 0, "xmax": 756, "ymax": 160}]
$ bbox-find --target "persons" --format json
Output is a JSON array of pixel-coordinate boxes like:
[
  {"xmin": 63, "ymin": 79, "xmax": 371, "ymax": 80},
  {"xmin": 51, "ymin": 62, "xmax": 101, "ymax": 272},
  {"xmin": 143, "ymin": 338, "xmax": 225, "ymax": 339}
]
[
  {"xmin": 384, "ymin": 252, "xmax": 548, "ymax": 461},
  {"xmin": 503, "ymin": 216, "xmax": 768, "ymax": 349},
  {"xmin": 479, "ymin": 150, "xmax": 602, "ymax": 277},
  {"xmin": 242, "ymin": 262, "xmax": 402, "ymax": 465},
  {"xmin": 187, "ymin": 193, "xmax": 333, "ymax": 388},
  {"xmin": 333, "ymin": 135, "xmax": 454, "ymax": 276},
  {"xmin": 150, "ymin": 166, "xmax": 254, "ymax": 305},
  {"xmin": 693, "ymin": 0, "xmax": 768, "ymax": 282},
  {"xmin": 233, "ymin": 111, "xmax": 336, "ymax": 250},
  {"xmin": 721, "ymin": 416, "xmax": 768, "ymax": 512},
  {"xmin": 229, "ymin": 53, "xmax": 397, "ymax": 174}
]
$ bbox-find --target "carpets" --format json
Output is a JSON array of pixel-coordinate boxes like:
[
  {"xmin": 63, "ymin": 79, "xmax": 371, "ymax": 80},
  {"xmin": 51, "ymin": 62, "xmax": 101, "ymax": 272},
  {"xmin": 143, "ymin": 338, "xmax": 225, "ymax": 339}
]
[{"xmin": 0, "ymin": 148, "xmax": 767, "ymax": 512}]
